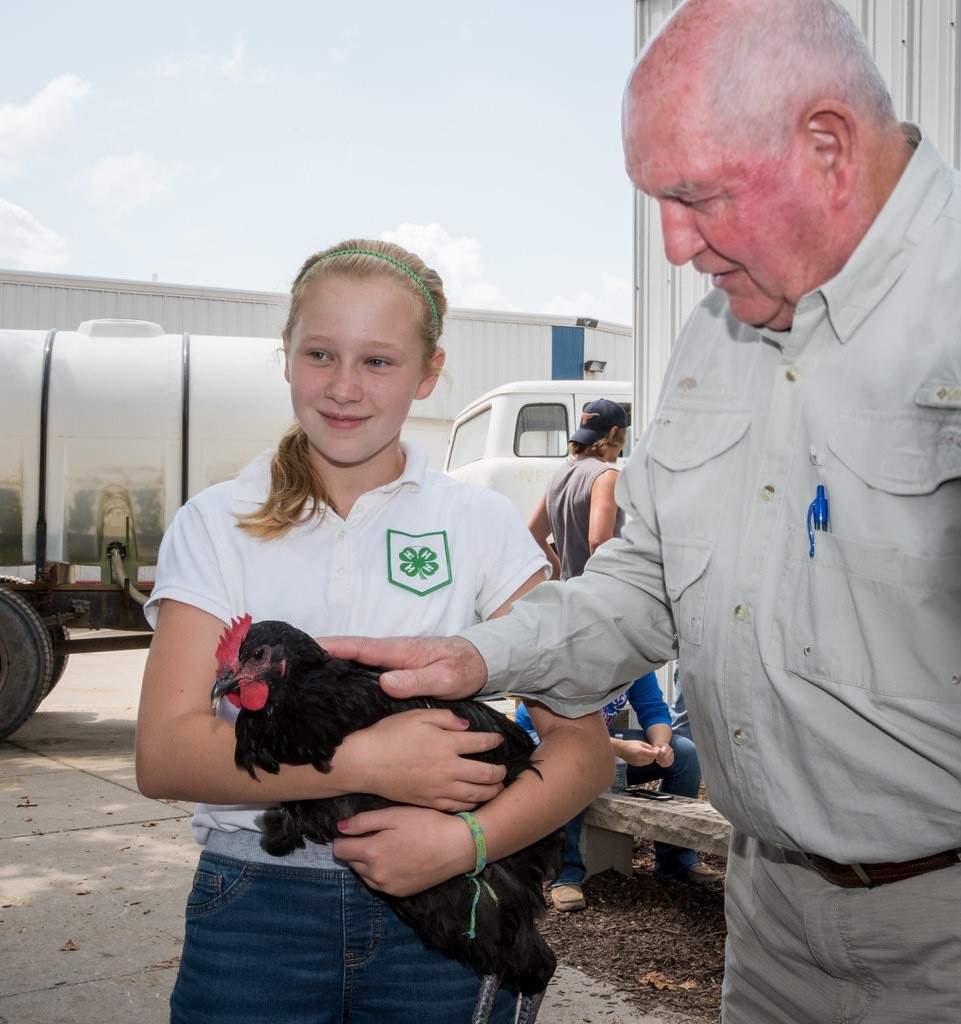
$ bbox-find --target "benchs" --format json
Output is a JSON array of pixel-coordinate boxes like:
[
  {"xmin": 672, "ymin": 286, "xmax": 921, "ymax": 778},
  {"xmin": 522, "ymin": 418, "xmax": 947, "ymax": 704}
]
[{"xmin": 583, "ymin": 789, "xmax": 732, "ymax": 858}]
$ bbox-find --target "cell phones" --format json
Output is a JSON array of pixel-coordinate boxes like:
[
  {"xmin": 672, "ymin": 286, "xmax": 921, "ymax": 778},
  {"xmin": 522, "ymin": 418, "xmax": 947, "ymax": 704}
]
[{"xmin": 624, "ymin": 787, "xmax": 673, "ymax": 801}]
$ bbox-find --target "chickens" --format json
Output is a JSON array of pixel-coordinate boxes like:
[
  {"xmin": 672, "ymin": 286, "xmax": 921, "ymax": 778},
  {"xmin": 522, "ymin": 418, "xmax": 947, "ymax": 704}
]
[{"xmin": 211, "ymin": 614, "xmax": 558, "ymax": 1024}]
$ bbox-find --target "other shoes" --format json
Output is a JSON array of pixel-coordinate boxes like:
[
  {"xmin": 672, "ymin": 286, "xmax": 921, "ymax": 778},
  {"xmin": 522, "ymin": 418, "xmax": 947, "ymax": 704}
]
[
  {"xmin": 678, "ymin": 862, "xmax": 722, "ymax": 882},
  {"xmin": 551, "ymin": 882, "xmax": 587, "ymax": 910}
]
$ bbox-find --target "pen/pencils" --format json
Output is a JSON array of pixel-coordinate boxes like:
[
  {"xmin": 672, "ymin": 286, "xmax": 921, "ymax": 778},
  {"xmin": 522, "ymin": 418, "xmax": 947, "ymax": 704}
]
[{"xmin": 806, "ymin": 484, "xmax": 830, "ymax": 557}]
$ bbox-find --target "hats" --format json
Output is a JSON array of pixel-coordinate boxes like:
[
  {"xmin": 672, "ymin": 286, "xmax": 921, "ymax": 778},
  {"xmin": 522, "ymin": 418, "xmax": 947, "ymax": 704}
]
[{"xmin": 568, "ymin": 398, "xmax": 626, "ymax": 446}]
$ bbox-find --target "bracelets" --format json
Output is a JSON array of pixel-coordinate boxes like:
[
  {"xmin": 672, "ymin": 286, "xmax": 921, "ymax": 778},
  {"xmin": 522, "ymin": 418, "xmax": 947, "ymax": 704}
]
[{"xmin": 455, "ymin": 805, "xmax": 496, "ymax": 938}]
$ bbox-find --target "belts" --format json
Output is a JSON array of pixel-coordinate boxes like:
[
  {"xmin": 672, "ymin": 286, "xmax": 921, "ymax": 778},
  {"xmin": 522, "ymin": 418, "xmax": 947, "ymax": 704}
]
[{"xmin": 731, "ymin": 828, "xmax": 960, "ymax": 886}]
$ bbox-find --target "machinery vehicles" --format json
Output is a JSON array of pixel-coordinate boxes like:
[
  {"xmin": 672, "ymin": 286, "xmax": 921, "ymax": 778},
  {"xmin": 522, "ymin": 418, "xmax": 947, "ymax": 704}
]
[{"xmin": 1, "ymin": 267, "xmax": 636, "ymax": 743}]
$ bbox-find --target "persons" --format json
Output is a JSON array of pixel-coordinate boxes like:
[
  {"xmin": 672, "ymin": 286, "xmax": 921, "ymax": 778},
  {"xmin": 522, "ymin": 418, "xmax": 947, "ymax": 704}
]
[
  {"xmin": 516, "ymin": 398, "xmax": 729, "ymax": 908},
  {"xmin": 315, "ymin": 0, "xmax": 960, "ymax": 1024},
  {"xmin": 135, "ymin": 238, "xmax": 617, "ymax": 1024}
]
